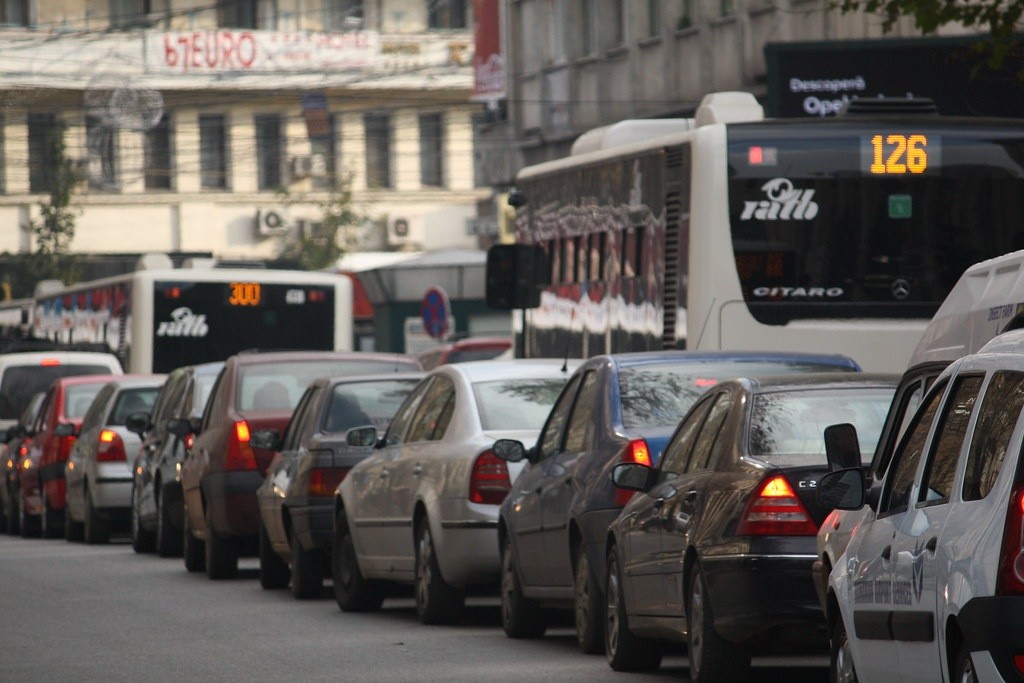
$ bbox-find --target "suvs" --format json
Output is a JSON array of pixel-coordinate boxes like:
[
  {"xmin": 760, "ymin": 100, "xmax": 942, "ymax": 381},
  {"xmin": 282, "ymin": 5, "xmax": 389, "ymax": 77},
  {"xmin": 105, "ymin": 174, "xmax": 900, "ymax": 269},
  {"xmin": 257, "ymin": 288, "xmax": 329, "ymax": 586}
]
[
  {"xmin": 811, "ymin": 307, "xmax": 1024, "ymax": 683},
  {"xmin": 166, "ymin": 350, "xmax": 429, "ymax": 578}
]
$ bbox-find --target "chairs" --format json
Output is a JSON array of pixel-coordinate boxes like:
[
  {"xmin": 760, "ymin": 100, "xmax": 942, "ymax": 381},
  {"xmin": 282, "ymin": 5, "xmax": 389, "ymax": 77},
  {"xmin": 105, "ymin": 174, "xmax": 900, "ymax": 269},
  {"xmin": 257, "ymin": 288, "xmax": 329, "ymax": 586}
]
[
  {"xmin": 254, "ymin": 381, "xmax": 291, "ymax": 410},
  {"xmin": 119, "ymin": 397, "xmax": 146, "ymax": 423},
  {"xmin": 75, "ymin": 398, "xmax": 92, "ymax": 419}
]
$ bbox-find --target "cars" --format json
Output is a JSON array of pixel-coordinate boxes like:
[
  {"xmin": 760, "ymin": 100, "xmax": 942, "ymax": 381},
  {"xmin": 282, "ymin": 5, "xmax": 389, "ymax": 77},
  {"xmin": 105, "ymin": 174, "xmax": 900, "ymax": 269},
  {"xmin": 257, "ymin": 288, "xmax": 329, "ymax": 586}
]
[
  {"xmin": 417, "ymin": 337, "xmax": 514, "ymax": 371},
  {"xmin": 52, "ymin": 374, "xmax": 172, "ymax": 545},
  {"xmin": 125, "ymin": 359, "xmax": 291, "ymax": 558},
  {"xmin": 251, "ymin": 367, "xmax": 438, "ymax": 598},
  {"xmin": 330, "ymin": 355, "xmax": 593, "ymax": 627},
  {"xmin": 602, "ymin": 369, "xmax": 905, "ymax": 683},
  {"xmin": 0, "ymin": 372, "xmax": 161, "ymax": 541},
  {"xmin": 490, "ymin": 347, "xmax": 865, "ymax": 654}
]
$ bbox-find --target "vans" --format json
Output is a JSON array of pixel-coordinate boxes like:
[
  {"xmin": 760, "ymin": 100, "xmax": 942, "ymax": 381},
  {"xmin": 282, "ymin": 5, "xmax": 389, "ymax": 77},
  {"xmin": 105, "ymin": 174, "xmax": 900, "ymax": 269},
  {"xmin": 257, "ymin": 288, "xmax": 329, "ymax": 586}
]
[
  {"xmin": 814, "ymin": 246, "xmax": 1024, "ymax": 645},
  {"xmin": 0, "ymin": 350, "xmax": 126, "ymax": 450}
]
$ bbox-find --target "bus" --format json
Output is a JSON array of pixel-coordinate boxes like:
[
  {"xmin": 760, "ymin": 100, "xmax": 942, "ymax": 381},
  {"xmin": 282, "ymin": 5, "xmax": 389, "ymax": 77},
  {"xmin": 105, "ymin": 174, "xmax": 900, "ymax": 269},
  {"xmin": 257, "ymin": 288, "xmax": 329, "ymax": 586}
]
[
  {"xmin": 485, "ymin": 90, "xmax": 1024, "ymax": 383},
  {"xmin": 18, "ymin": 248, "xmax": 355, "ymax": 375}
]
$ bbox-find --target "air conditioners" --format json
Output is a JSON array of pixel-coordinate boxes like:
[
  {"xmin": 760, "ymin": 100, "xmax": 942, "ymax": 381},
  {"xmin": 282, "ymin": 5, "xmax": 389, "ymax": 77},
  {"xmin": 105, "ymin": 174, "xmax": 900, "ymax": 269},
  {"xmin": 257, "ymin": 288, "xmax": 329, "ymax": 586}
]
[
  {"xmin": 259, "ymin": 208, "xmax": 290, "ymax": 236},
  {"xmin": 385, "ymin": 214, "xmax": 425, "ymax": 245},
  {"xmin": 292, "ymin": 153, "xmax": 325, "ymax": 179}
]
